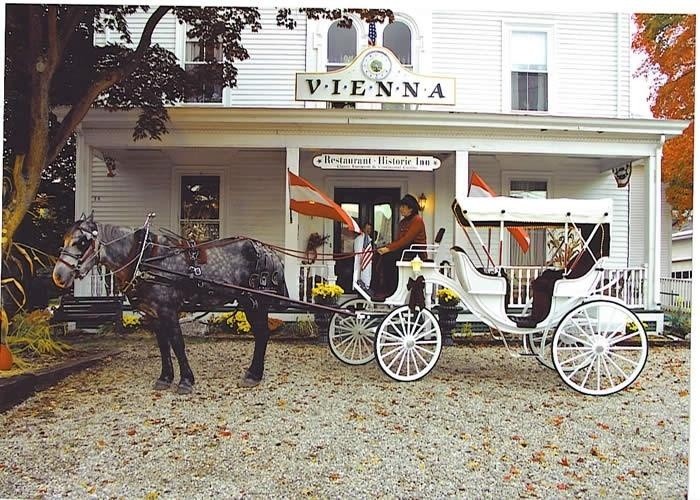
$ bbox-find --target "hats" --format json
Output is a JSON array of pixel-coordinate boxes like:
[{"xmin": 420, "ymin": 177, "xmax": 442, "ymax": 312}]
[{"xmin": 403, "ymin": 194, "xmax": 419, "ymax": 209}]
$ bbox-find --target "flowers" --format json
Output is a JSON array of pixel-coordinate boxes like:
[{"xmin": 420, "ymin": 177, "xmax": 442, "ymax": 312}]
[
  {"xmin": 311, "ymin": 282, "xmax": 344, "ymax": 300},
  {"xmin": 436, "ymin": 288, "xmax": 461, "ymax": 306}
]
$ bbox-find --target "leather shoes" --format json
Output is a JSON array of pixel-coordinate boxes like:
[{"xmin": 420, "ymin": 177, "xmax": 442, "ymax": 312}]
[{"xmin": 372, "ymin": 291, "xmax": 386, "ymax": 301}]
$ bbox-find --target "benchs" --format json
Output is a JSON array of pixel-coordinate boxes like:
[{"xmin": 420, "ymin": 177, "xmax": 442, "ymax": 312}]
[{"xmin": 51, "ymin": 295, "xmax": 126, "ymax": 324}]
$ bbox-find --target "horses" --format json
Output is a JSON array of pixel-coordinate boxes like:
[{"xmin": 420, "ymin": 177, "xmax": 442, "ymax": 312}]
[{"xmin": 53, "ymin": 211, "xmax": 287, "ymax": 396}]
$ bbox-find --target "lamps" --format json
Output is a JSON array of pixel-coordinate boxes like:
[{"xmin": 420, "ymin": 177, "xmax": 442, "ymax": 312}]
[{"xmin": 418, "ymin": 193, "xmax": 427, "ymax": 211}]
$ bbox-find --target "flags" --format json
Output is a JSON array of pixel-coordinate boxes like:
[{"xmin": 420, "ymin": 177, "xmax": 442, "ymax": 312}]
[
  {"xmin": 289, "ymin": 169, "xmax": 361, "ymax": 233},
  {"xmin": 468, "ymin": 174, "xmax": 531, "ymax": 255},
  {"xmin": 361, "ymin": 234, "xmax": 374, "ymax": 271}
]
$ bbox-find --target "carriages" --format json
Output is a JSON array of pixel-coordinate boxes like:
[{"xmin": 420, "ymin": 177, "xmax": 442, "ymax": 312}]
[{"xmin": 50, "ymin": 190, "xmax": 654, "ymax": 399}]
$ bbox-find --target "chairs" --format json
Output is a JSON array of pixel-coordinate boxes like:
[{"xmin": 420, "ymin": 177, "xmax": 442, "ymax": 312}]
[
  {"xmin": 402, "ymin": 228, "xmax": 446, "ymax": 260},
  {"xmin": 450, "ymin": 245, "xmax": 518, "ymax": 326},
  {"xmin": 532, "ymin": 252, "xmax": 601, "ymax": 324}
]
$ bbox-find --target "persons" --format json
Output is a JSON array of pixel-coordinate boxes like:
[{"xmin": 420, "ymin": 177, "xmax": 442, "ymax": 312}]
[
  {"xmin": 351, "ymin": 223, "xmax": 374, "ymax": 292},
  {"xmin": 356, "ymin": 192, "xmax": 427, "ymax": 302}
]
